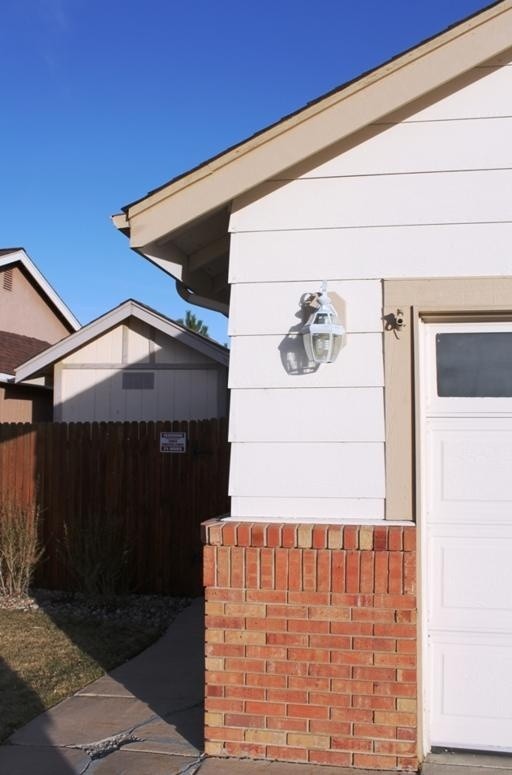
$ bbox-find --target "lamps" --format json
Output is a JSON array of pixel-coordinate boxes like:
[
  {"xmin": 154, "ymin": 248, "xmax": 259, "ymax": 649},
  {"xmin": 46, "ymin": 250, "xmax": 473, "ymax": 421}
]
[{"xmin": 297, "ymin": 276, "xmax": 346, "ymax": 366}]
